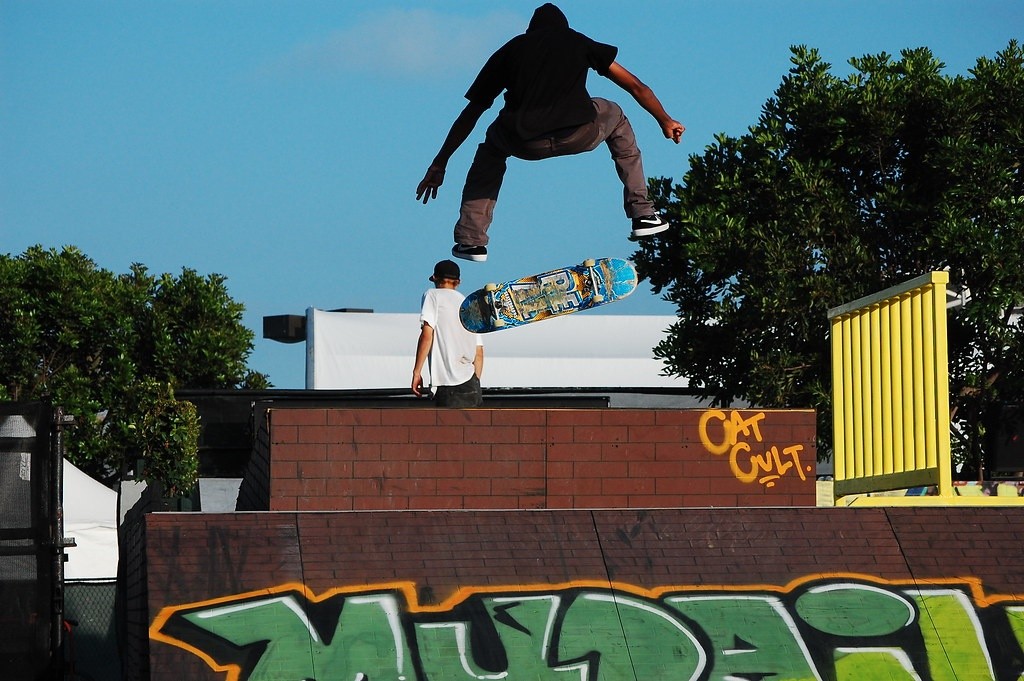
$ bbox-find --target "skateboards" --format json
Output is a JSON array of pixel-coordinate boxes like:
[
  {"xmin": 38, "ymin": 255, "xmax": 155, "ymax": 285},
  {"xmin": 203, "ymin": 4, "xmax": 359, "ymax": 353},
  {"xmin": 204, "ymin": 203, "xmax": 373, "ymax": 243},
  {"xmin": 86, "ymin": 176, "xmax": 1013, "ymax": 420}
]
[{"xmin": 459, "ymin": 256, "xmax": 638, "ymax": 334}]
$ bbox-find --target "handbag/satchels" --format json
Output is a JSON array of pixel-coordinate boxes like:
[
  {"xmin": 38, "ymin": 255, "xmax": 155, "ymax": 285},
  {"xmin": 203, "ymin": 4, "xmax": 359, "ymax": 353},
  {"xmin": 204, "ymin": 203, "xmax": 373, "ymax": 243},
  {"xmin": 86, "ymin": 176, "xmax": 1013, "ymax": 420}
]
[{"xmin": 442, "ymin": 376, "xmax": 480, "ymax": 409}]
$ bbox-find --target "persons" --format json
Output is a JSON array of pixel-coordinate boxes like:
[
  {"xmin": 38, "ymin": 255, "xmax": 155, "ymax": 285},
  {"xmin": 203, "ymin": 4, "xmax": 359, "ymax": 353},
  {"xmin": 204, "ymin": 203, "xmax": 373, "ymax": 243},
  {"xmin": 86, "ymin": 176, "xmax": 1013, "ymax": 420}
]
[
  {"xmin": 416, "ymin": 3, "xmax": 686, "ymax": 262},
  {"xmin": 411, "ymin": 259, "xmax": 483, "ymax": 409}
]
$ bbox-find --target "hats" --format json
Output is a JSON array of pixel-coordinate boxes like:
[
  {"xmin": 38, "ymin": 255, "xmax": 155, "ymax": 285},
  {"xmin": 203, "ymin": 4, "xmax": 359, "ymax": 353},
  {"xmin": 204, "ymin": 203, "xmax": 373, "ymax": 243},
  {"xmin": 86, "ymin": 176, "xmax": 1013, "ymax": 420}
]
[
  {"xmin": 428, "ymin": 260, "xmax": 461, "ymax": 281},
  {"xmin": 527, "ymin": 2, "xmax": 569, "ymax": 37}
]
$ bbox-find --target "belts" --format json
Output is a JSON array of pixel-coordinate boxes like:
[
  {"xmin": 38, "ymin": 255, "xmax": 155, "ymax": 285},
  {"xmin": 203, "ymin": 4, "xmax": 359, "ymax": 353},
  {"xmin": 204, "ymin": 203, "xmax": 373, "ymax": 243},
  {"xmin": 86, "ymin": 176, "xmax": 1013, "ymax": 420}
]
[{"xmin": 528, "ymin": 121, "xmax": 594, "ymax": 149}]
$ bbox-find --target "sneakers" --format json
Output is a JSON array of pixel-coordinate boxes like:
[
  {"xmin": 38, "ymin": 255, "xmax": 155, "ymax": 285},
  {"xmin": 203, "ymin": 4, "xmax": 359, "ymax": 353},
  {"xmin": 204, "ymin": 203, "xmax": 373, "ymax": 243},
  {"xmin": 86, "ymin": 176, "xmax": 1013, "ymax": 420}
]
[
  {"xmin": 451, "ymin": 243, "xmax": 488, "ymax": 262},
  {"xmin": 631, "ymin": 213, "xmax": 669, "ymax": 237}
]
